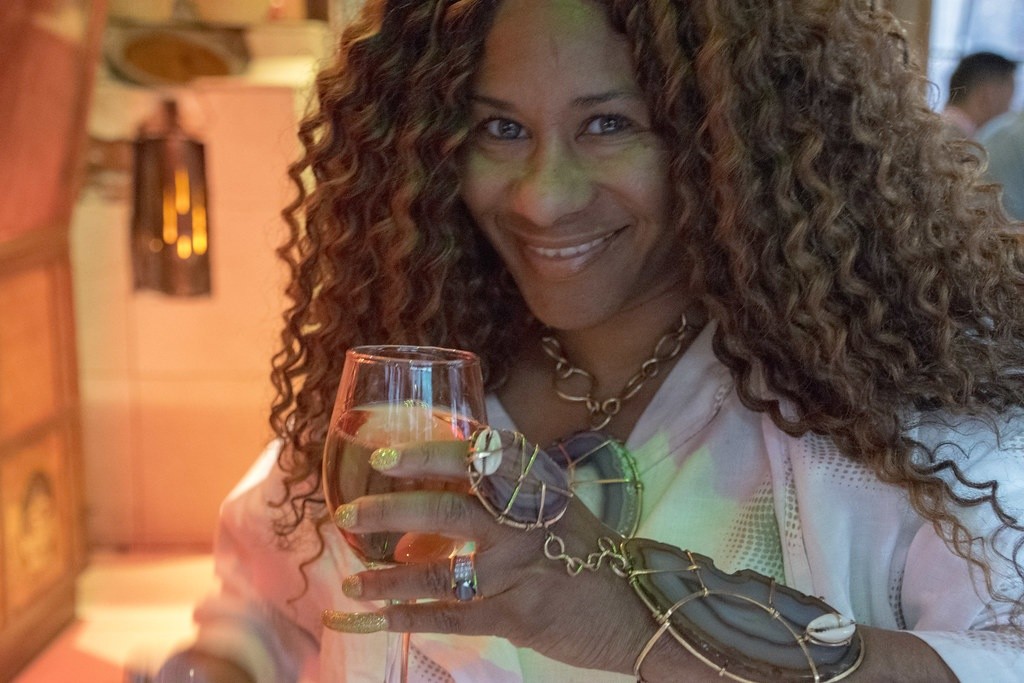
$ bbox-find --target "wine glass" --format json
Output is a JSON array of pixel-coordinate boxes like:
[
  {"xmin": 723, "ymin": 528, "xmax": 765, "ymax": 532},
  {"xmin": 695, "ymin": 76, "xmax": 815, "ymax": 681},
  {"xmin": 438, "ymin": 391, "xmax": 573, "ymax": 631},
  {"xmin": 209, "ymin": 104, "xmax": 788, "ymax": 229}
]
[{"xmin": 322, "ymin": 345, "xmax": 489, "ymax": 683}]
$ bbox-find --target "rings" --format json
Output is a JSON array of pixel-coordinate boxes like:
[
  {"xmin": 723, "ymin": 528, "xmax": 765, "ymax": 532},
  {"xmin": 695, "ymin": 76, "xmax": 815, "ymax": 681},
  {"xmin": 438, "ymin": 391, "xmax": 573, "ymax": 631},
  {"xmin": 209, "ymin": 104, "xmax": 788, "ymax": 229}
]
[{"xmin": 453, "ymin": 545, "xmax": 477, "ymax": 601}]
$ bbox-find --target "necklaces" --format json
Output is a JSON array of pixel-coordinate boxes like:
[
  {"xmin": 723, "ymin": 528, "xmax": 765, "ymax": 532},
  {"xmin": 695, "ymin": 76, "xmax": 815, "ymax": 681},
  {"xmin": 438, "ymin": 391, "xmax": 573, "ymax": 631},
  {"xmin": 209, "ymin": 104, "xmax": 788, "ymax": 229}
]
[{"xmin": 466, "ymin": 297, "xmax": 714, "ymax": 562}]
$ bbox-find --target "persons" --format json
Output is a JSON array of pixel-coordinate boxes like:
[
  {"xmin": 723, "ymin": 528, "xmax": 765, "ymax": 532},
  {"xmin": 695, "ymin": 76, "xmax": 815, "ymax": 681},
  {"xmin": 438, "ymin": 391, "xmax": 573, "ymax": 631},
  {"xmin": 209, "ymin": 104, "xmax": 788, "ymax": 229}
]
[
  {"xmin": 939, "ymin": 51, "xmax": 1023, "ymax": 137},
  {"xmin": 156, "ymin": 1, "xmax": 1021, "ymax": 682}
]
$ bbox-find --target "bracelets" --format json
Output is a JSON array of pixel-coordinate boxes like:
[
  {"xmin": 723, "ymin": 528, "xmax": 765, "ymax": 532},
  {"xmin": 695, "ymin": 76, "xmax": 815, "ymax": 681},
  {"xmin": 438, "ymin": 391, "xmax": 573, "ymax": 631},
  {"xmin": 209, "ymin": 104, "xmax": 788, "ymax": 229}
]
[{"xmin": 469, "ymin": 424, "xmax": 866, "ymax": 682}]
